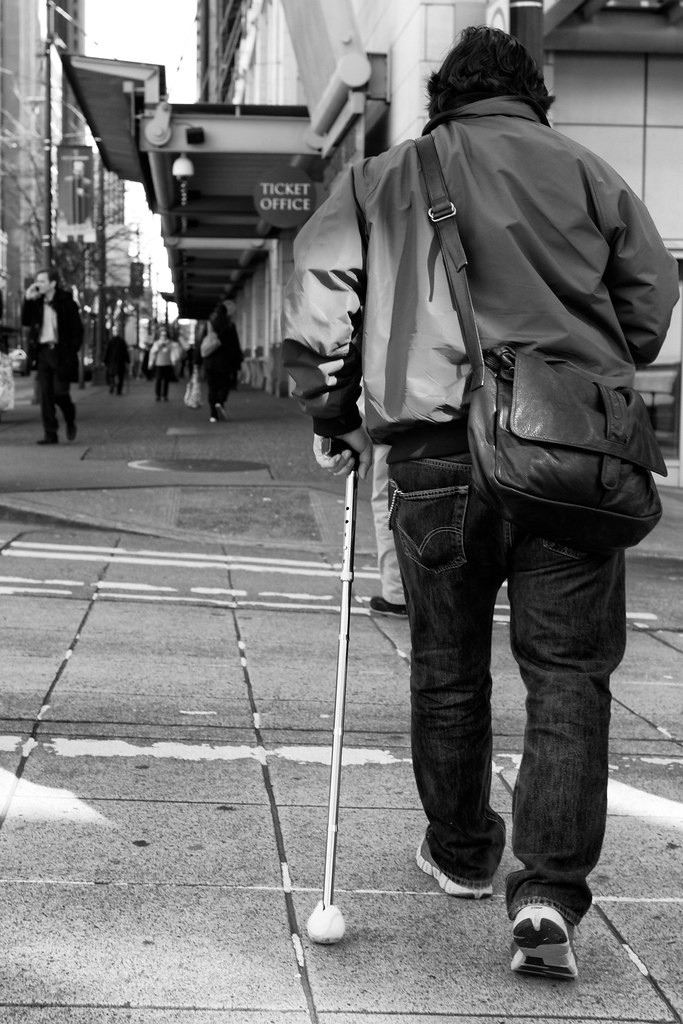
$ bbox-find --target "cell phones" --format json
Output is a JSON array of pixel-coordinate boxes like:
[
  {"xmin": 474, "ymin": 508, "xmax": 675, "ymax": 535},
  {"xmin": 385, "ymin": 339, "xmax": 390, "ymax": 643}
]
[{"xmin": 34, "ymin": 285, "xmax": 39, "ymax": 292}]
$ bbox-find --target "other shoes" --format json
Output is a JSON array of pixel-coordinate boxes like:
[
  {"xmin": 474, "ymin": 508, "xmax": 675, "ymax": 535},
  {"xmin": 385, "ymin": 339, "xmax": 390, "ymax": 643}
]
[
  {"xmin": 66, "ymin": 419, "xmax": 77, "ymax": 440},
  {"xmin": 370, "ymin": 596, "xmax": 409, "ymax": 617},
  {"xmin": 156, "ymin": 397, "xmax": 161, "ymax": 401},
  {"xmin": 215, "ymin": 403, "xmax": 227, "ymax": 421},
  {"xmin": 164, "ymin": 397, "xmax": 168, "ymax": 401},
  {"xmin": 37, "ymin": 434, "xmax": 58, "ymax": 445},
  {"xmin": 209, "ymin": 417, "xmax": 217, "ymax": 423}
]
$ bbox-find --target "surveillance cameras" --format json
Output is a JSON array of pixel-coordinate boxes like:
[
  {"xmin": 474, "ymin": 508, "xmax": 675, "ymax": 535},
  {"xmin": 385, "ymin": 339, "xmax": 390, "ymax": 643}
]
[{"xmin": 172, "ymin": 156, "xmax": 194, "ymax": 181}]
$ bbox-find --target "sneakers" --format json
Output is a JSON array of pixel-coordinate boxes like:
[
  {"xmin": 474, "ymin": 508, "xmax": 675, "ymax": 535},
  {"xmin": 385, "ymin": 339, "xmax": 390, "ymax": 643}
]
[
  {"xmin": 511, "ymin": 905, "xmax": 579, "ymax": 981},
  {"xmin": 416, "ymin": 836, "xmax": 493, "ymax": 899}
]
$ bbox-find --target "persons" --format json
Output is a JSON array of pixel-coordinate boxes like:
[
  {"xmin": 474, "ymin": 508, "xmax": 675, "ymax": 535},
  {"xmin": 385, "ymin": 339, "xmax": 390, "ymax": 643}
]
[
  {"xmin": 21, "ymin": 270, "xmax": 83, "ymax": 444},
  {"xmin": 193, "ymin": 306, "xmax": 244, "ymax": 422},
  {"xmin": 283, "ymin": 25, "xmax": 681, "ymax": 977},
  {"xmin": 131, "ymin": 331, "xmax": 193, "ymax": 402},
  {"xmin": 103, "ymin": 333, "xmax": 131, "ymax": 395}
]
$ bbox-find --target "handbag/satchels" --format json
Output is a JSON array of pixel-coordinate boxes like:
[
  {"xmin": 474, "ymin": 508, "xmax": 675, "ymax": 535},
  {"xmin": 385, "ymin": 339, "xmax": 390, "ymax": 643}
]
[
  {"xmin": 200, "ymin": 321, "xmax": 222, "ymax": 358},
  {"xmin": 0, "ymin": 350, "xmax": 14, "ymax": 412},
  {"xmin": 184, "ymin": 365, "xmax": 204, "ymax": 409},
  {"xmin": 467, "ymin": 345, "xmax": 667, "ymax": 547}
]
